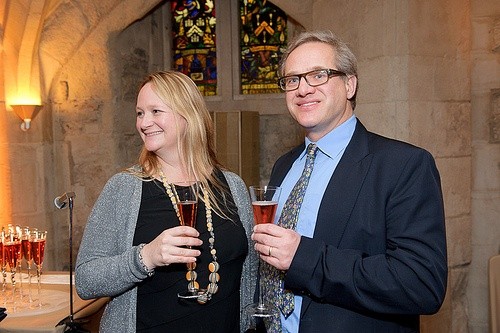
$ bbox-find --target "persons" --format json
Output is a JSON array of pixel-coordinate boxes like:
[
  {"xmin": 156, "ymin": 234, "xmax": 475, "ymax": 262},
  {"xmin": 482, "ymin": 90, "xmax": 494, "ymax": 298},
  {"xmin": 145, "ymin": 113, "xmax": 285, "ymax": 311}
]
[
  {"xmin": 251, "ymin": 30, "xmax": 447, "ymax": 333},
  {"xmin": 74, "ymin": 70, "xmax": 260, "ymax": 333}
]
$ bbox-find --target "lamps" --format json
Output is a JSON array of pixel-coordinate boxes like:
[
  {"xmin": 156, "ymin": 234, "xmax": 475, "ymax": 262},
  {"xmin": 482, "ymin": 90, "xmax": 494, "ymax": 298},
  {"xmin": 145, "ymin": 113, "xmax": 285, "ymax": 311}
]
[{"xmin": 9, "ymin": 100, "xmax": 43, "ymax": 131}]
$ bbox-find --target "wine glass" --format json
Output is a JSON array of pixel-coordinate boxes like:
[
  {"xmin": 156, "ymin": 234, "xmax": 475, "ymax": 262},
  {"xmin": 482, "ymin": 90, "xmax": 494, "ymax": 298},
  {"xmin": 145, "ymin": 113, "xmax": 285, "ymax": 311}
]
[
  {"xmin": 171, "ymin": 181, "xmax": 209, "ymax": 298},
  {"xmin": 0, "ymin": 222, "xmax": 50, "ymax": 313},
  {"xmin": 245, "ymin": 185, "xmax": 283, "ymax": 317}
]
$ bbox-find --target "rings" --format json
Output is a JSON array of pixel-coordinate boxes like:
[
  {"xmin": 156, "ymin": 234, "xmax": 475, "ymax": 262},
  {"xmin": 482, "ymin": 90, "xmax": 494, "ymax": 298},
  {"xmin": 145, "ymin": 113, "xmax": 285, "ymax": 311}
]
[{"xmin": 269, "ymin": 246, "xmax": 272, "ymax": 256}]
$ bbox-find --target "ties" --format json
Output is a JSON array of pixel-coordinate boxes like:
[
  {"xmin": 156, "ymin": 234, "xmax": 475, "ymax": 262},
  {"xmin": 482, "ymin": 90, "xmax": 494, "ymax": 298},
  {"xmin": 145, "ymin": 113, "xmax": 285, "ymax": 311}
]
[{"xmin": 262, "ymin": 142, "xmax": 318, "ymax": 333}]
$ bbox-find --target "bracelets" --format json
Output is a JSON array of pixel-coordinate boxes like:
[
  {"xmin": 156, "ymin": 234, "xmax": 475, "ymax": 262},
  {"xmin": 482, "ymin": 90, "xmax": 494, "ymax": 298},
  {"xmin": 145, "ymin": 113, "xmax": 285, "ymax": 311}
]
[{"xmin": 139, "ymin": 244, "xmax": 155, "ymax": 276}]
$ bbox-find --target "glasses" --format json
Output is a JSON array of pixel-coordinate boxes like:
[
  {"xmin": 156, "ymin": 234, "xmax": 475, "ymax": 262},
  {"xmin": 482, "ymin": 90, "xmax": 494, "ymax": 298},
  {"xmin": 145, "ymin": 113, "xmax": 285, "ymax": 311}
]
[{"xmin": 277, "ymin": 68, "xmax": 347, "ymax": 91}]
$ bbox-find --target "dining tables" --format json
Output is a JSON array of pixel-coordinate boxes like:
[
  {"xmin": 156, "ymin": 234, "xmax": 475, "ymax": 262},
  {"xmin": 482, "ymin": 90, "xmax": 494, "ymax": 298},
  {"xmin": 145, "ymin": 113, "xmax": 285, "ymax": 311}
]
[{"xmin": 0, "ymin": 268, "xmax": 111, "ymax": 333}]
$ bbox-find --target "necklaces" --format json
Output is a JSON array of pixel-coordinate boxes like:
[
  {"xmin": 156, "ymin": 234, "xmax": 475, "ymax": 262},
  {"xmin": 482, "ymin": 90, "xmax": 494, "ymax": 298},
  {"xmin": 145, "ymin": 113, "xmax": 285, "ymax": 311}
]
[{"xmin": 154, "ymin": 155, "xmax": 220, "ymax": 303}]
